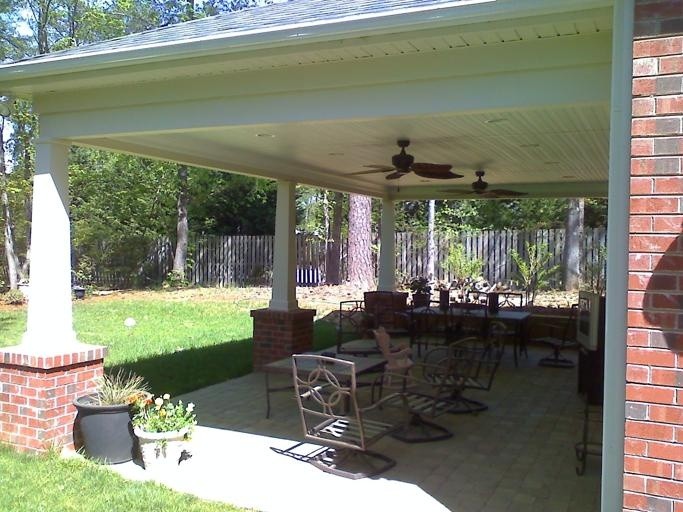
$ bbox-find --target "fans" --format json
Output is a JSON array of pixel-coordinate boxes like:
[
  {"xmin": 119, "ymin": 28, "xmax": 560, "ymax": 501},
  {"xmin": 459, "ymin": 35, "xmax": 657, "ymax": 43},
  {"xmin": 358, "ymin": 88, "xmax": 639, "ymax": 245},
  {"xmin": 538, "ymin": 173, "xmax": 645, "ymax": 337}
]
[
  {"xmin": 346, "ymin": 138, "xmax": 464, "ymax": 181},
  {"xmin": 439, "ymin": 170, "xmax": 527, "ymax": 201}
]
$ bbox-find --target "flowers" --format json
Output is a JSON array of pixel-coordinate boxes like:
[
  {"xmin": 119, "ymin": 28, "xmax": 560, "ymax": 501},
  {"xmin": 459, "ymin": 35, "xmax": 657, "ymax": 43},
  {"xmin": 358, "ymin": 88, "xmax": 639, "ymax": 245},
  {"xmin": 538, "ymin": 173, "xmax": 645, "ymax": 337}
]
[{"xmin": 131, "ymin": 394, "xmax": 198, "ymax": 440}]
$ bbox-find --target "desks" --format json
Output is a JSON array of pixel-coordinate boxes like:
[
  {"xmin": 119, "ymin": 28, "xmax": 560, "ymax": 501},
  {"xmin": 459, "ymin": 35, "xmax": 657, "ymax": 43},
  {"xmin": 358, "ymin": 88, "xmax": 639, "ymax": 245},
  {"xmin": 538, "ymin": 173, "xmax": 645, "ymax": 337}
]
[
  {"xmin": 573, "ymin": 349, "xmax": 604, "ymax": 476},
  {"xmin": 260, "ymin": 354, "xmax": 389, "ymax": 419}
]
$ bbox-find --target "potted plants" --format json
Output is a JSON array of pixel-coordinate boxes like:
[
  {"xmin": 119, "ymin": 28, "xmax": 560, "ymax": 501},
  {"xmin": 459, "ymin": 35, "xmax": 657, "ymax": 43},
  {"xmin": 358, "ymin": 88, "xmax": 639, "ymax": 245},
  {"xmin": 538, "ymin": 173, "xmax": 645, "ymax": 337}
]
[{"xmin": 409, "ymin": 275, "xmax": 431, "ymax": 308}]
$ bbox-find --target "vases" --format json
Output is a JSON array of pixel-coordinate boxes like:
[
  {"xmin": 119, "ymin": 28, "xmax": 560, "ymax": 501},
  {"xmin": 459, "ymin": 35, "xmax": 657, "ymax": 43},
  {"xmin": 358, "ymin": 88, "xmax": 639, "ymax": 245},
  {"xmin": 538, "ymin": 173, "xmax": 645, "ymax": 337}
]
[
  {"xmin": 135, "ymin": 432, "xmax": 187, "ymax": 474},
  {"xmin": 72, "ymin": 364, "xmax": 154, "ymax": 464}
]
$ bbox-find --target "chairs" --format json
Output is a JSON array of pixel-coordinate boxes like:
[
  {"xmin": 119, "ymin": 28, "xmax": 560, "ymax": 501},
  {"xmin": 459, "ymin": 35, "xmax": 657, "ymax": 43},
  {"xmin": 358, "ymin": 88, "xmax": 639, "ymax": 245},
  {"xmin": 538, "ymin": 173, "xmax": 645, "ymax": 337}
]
[
  {"xmin": 292, "ymin": 354, "xmax": 409, "ymax": 478},
  {"xmin": 530, "ymin": 304, "xmax": 579, "ymax": 368},
  {"xmin": 337, "ymin": 289, "xmax": 531, "ymax": 444}
]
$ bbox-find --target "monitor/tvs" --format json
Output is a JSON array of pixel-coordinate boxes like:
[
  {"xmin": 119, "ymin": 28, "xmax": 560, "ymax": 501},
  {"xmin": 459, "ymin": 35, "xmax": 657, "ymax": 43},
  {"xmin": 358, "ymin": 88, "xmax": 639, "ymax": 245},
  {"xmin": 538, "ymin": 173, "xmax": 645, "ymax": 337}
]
[{"xmin": 576, "ymin": 291, "xmax": 605, "ymax": 352}]
